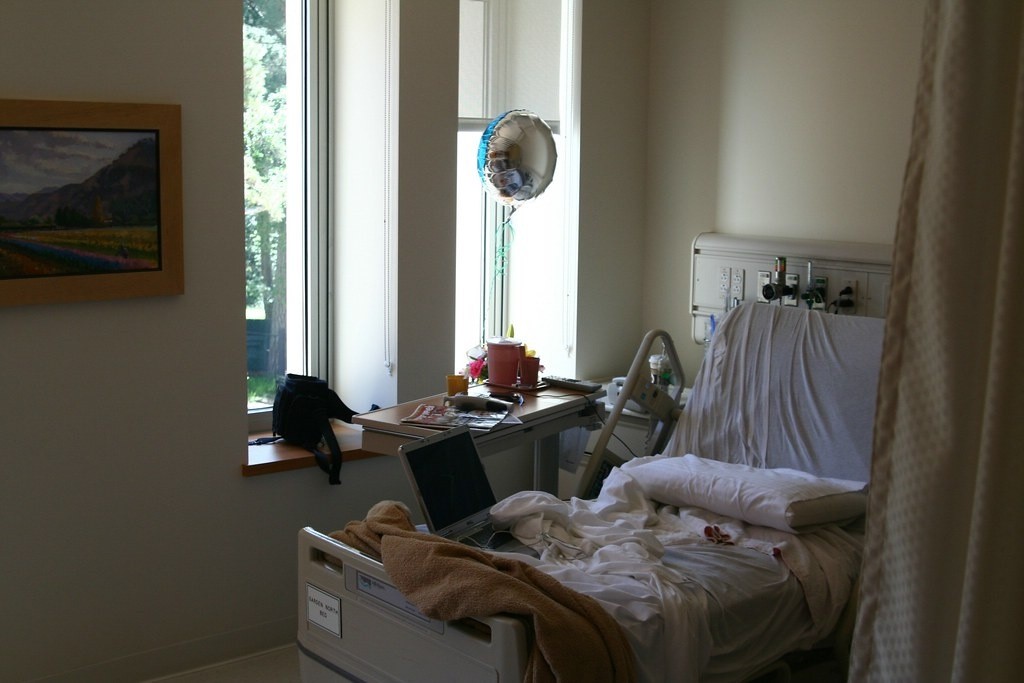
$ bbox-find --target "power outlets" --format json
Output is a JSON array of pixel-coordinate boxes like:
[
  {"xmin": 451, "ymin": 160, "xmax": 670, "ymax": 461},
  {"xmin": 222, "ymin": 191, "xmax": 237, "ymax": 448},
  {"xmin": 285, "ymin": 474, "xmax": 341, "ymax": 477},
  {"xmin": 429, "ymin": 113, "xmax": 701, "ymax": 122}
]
[{"xmin": 720, "ymin": 266, "xmax": 744, "ymax": 301}]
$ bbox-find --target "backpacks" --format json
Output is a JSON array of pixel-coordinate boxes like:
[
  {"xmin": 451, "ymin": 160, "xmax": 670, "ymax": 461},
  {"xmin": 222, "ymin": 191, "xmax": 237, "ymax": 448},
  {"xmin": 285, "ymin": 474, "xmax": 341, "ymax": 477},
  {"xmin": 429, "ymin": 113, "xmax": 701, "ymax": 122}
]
[{"xmin": 272, "ymin": 373, "xmax": 382, "ymax": 486}]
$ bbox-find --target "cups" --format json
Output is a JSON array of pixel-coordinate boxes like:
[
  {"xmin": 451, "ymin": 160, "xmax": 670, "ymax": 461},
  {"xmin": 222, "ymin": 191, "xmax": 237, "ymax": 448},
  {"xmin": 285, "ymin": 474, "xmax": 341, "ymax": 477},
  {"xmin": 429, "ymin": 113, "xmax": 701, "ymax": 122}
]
[
  {"xmin": 485, "ymin": 336, "xmax": 521, "ymax": 385},
  {"xmin": 445, "ymin": 375, "xmax": 468, "ymax": 397},
  {"xmin": 520, "ymin": 357, "xmax": 540, "ymax": 387},
  {"xmin": 648, "ymin": 353, "xmax": 672, "ymax": 397}
]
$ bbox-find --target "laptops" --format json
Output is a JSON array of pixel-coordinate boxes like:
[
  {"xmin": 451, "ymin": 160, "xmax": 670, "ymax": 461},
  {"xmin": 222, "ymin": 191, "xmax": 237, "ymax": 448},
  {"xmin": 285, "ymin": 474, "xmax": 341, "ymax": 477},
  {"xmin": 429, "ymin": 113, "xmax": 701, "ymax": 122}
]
[{"xmin": 398, "ymin": 425, "xmax": 544, "ymax": 560}]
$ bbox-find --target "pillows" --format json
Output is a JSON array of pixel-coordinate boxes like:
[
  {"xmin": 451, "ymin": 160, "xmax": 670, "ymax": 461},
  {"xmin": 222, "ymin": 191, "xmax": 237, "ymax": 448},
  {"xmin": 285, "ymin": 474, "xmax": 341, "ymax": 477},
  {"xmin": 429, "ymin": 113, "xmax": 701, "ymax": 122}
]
[{"xmin": 616, "ymin": 453, "xmax": 878, "ymax": 536}]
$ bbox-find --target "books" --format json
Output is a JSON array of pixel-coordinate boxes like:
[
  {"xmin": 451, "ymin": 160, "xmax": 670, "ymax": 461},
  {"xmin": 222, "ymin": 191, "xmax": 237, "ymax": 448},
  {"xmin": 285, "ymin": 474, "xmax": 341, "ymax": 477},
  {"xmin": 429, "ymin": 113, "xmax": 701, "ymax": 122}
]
[{"xmin": 402, "ymin": 403, "xmax": 510, "ymax": 432}]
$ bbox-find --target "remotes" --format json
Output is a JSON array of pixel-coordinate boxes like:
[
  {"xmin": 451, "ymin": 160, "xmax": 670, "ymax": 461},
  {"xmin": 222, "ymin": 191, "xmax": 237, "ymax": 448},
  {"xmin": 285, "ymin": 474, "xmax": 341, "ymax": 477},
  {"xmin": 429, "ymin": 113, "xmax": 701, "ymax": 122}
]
[{"xmin": 542, "ymin": 376, "xmax": 602, "ymax": 392}]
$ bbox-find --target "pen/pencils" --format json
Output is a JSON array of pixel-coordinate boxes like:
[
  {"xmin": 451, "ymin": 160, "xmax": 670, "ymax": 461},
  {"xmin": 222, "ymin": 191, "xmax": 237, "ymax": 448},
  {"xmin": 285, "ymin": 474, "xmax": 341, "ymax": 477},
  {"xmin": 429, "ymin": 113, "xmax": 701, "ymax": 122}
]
[{"xmin": 459, "ymin": 415, "xmax": 501, "ymax": 420}]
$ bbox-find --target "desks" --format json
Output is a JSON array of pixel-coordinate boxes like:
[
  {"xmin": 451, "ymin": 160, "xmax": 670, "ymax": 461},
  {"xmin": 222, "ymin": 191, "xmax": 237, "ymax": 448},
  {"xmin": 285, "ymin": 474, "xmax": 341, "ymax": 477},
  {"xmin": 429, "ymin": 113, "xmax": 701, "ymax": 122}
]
[{"xmin": 351, "ymin": 373, "xmax": 607, "ymax": 520}]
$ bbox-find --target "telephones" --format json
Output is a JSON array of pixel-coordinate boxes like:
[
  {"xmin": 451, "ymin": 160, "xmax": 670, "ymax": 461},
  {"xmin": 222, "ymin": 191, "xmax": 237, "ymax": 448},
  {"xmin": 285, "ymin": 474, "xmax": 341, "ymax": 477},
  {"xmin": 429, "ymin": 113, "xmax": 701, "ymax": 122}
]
[{"xmin": 608, "ymin": 377, "xmax": 649, "ymax": 414}]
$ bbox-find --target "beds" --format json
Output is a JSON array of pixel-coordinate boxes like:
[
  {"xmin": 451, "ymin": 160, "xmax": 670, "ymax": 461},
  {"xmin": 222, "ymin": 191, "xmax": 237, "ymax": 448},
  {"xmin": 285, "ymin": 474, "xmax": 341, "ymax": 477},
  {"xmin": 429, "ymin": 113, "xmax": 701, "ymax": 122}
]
[{"xmin": 292, "ymin": 304, "xmax": 887, "ymax": 681}]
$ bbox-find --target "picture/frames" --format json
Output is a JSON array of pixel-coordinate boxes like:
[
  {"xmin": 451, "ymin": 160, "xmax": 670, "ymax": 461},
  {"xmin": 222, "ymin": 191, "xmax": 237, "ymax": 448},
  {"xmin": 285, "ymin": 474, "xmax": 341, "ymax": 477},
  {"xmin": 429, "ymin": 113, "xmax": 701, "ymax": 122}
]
[{"xmin": 0, "ymin": 99, "xmax": 182, "ymax": 308}]
